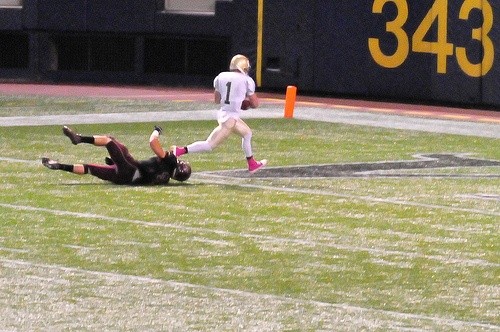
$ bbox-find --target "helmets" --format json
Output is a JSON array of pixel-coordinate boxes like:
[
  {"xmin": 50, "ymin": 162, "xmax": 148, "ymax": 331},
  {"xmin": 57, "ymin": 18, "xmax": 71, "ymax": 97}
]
[
  {"xmin": 229, "ymin": 54, "xmax": 251, "ymax": 75},
  {"xmin": 172, "ymin": 159, "xmax": 191, "ymax": 181}
]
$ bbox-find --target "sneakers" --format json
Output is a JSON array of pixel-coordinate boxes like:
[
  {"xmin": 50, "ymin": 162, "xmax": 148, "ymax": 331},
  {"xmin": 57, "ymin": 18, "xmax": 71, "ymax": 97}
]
[
  {"xmin": 42, "ymin": 157, "xmax": 60, "ymax": 170},
  {"xmin": 63, "ymin": 126, "xmax": 82, "ymax": 145}
]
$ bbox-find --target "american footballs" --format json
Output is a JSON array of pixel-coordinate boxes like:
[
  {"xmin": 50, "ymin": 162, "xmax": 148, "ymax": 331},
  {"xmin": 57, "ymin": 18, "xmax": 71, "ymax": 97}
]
[{"xmin": 241, "ymin": 100, "xmax": 250, "ymax": 110}]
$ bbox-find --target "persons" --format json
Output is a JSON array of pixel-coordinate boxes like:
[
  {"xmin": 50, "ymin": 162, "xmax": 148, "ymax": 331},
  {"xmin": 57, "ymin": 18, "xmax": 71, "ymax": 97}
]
[
  {"xmin": 41, "ymin": 124, "xmax": 193, "ymax": 186},
  {"xmin": 170, "ymin": 54, "xmax": 268, "ymax": 178}
]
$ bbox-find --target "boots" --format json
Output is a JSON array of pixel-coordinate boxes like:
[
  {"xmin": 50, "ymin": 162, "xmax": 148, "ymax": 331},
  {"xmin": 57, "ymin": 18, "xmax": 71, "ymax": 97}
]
[
  {"xmin": 246, "ymin": 156, "xmax": 267, "ymax": 174},
  {"xmin": 172, "ymin": 145, "xmax": 188, "ymax": 157}
]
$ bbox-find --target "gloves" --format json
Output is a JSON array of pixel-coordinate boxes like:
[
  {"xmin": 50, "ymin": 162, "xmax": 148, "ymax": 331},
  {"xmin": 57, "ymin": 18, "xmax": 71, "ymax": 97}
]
[
  {"xmin": 155, "ymin": 125, "xmax": 162, "ymax": 134},
  {"xmin": 105, "ymin": 157, "xmax": 114, "ymax": 165}
]
